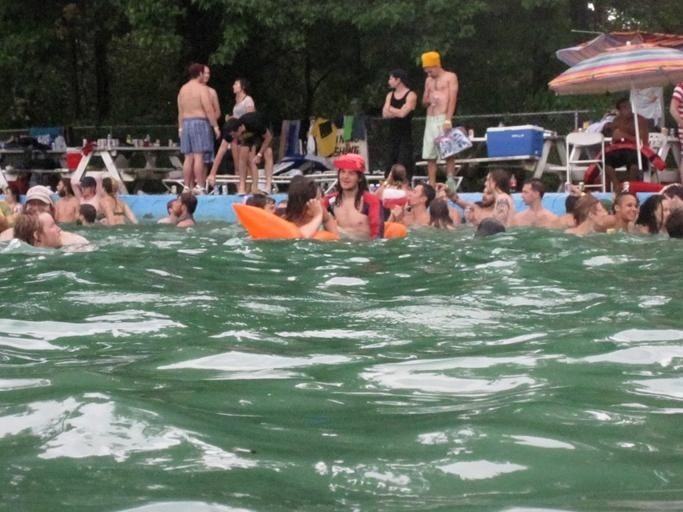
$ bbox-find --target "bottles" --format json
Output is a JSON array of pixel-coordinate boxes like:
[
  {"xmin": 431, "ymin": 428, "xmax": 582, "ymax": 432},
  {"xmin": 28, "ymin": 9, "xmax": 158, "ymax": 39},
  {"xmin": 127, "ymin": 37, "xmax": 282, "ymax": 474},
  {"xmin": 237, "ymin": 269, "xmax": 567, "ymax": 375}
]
[
  {"xmin": 446, "ymin": 172, "xmax": 456, "ymax": 193},
  {"xmin": 509, "ymin": 174, "xmax": 518, "ymax": 192},
  {"xmin": 213, "ymin": 183, "xmax": 228, "ymax": 195}
]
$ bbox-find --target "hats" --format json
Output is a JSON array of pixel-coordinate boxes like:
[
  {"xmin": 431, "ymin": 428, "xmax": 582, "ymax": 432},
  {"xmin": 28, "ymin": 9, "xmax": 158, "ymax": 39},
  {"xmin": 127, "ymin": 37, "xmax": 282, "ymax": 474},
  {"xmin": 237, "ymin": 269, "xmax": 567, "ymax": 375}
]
[
  {"xmin": 334, "ymin": 153, "xmax": 365, "ymax": 172},
  {"xmin": 421, "ymin": 51, "xmax": 441, "ymax": 68},
  {"xmin": 26, "ymin": 184, "xmax": 57, "ymax": 205}
]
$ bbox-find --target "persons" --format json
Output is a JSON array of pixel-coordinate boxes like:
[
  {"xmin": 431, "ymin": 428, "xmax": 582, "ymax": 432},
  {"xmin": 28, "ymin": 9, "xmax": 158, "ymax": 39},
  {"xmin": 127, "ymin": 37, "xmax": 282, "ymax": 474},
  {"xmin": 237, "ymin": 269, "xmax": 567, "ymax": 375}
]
[
  {"xmin": 248, "ymin": 153, "xmax": 383, "ymax": 239},
  {"xmin": 201, "ymin": 65, "xmax": 222, "ymax": 180},
  {"xmin": 0, "ymin": 176, "xmax": 137, "ymax": 249},
  {"xmin": 669, "ymin": 81, "xmax": 683, "ymax": 186},
  {"xmin": 205, "ymin": 111, "xmax": 274, "ymax": 196},
  {"xmin": 381, "ymin": 69, "xmax": 418, "ymax": 187},
  {"xmin": 226, "ymin": 78, "xmax": 256, "ymax": 191},
  {"xmin": 602, "ymin": 97, "xmax": 651, "ymax": 193},
  {"xmin": 511, "ymin": 177, "xmax": 683, "ymax": 238},
  {"xmin": 177, "ymin": 63, "xmax": 220, "ymax": 195},
  {"xmin": 375, "ymin": 164, "xmax": 514, "ymax": 236},
  {"xmin": 158, "ymin": 194, "xmax": 198, "ymax": 227},
  {"xmin": 420, "ymin": 51, "xmax": 459, "ymax": 190}
]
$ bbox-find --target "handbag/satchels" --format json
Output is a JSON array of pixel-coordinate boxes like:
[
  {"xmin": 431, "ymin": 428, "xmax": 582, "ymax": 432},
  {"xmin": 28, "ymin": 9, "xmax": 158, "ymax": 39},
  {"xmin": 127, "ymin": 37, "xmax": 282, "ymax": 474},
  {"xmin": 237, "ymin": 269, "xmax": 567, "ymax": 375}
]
[{"xmin": 434, "ymin": 125, "xmax": 473, "ymax": 160}]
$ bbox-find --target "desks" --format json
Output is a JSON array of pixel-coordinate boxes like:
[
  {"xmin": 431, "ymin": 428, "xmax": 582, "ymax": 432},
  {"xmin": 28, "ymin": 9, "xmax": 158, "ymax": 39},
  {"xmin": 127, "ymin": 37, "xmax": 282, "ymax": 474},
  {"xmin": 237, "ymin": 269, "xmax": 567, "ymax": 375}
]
[{"xmin": 60, "ymin": 135, "xmax": 183, "ymax": 196}]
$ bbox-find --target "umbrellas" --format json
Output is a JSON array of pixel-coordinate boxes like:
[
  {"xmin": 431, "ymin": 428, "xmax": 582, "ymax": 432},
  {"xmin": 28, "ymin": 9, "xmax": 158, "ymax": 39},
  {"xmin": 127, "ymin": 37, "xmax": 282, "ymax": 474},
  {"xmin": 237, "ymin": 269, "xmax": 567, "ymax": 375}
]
[{"xmin": 546, "ymin": 39, "xmax": 683, "ymax": 171}]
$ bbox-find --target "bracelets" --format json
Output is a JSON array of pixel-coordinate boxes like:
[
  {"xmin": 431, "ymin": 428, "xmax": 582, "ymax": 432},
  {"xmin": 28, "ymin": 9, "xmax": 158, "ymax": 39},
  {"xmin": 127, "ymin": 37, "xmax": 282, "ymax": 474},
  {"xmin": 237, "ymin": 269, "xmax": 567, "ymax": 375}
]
[
  {"xmin": 444, "ymin": 120, "xmax": 452, "ymax": 124},
  {"xmin": 213, "ymin": 127, "xmax": 219, "ymax": 130},
  {"xmin": 178, "ymin": 128, "xmax": 182, "ymax": 132}
]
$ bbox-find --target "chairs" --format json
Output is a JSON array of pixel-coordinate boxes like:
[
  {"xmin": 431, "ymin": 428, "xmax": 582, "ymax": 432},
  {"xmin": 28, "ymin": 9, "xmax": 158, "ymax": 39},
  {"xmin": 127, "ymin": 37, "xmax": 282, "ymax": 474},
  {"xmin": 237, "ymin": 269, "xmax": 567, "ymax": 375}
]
[{"xmin": 161, "ymin": 130, "xmax": 683, "ymax": 196}]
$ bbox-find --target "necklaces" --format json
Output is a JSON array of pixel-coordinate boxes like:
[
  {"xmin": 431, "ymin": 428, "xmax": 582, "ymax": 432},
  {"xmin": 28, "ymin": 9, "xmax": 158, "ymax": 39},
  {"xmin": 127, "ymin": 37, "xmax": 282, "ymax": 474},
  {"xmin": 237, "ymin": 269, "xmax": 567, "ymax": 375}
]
[{"xmin": 234, "ymin": 96, "xmax": 248, "ymax": 104}]
